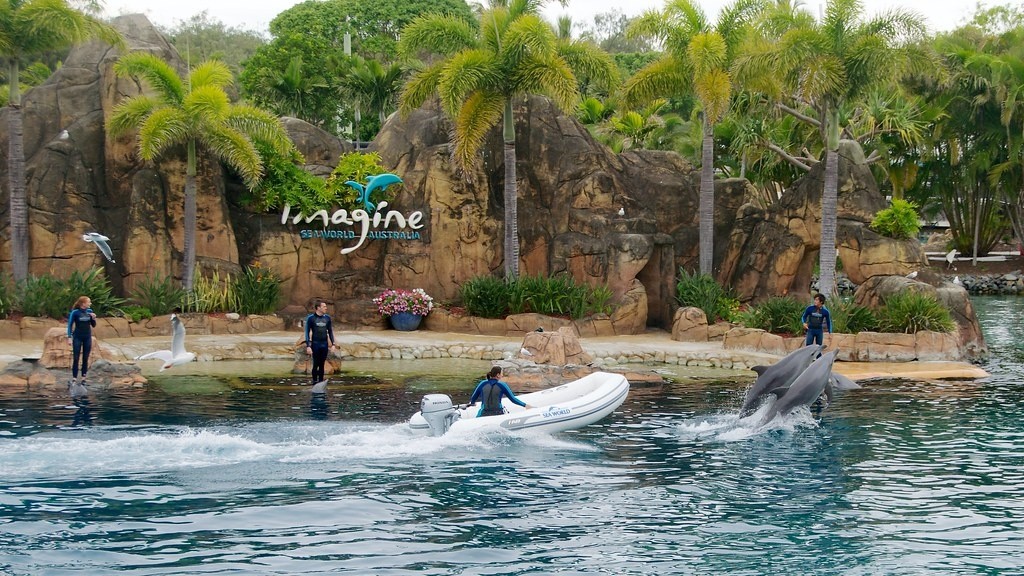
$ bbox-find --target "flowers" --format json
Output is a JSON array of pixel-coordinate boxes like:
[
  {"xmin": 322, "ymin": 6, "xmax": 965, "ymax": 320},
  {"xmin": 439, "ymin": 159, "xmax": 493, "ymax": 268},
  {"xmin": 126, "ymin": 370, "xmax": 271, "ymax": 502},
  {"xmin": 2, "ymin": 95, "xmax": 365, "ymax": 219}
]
[{"xmin": 373, "ymin": 288, "xmax": 434, "ymax": 316}]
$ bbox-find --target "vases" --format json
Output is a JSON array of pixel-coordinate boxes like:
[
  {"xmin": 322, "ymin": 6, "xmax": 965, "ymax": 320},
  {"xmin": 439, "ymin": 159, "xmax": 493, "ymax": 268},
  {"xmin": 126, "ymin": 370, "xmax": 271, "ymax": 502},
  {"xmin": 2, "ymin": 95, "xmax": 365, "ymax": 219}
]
[{"xmin": 389, "ymin": 311, "xmax": 422, "ymax": 331}]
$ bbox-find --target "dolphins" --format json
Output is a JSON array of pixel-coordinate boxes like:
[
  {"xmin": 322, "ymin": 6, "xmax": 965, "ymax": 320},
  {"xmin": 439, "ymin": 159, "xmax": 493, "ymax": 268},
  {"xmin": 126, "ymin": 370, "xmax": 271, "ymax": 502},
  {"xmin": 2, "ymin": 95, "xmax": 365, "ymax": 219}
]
[
  {"xmin": 312, "ymin": 378, "xmax": 331, "ymax": 393},
  {"xmin": 740, "ymin": 341, "xmax": 864, "ymax": 439}
]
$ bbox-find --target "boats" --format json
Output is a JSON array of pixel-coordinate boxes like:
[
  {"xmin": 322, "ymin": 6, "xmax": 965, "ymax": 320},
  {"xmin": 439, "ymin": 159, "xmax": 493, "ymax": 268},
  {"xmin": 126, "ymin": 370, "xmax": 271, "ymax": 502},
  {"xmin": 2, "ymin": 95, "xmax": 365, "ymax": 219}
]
[{"xmin": 405, "ymin": 371, "xmax": 630, "ymax": 449}]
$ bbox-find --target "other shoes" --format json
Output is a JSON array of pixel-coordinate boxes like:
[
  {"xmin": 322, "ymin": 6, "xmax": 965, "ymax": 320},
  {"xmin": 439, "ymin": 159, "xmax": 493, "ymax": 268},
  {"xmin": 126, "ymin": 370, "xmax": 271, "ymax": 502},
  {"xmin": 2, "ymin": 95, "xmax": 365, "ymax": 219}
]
[{"xmin": 81, "ymin": 380, "xmax": 90, "ymax": 386}]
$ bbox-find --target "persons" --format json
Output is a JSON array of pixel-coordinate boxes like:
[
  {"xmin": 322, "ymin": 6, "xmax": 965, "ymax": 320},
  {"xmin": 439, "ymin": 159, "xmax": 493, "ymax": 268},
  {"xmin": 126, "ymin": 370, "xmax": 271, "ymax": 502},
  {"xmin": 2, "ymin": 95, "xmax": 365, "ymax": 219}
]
[
  {"xmin": 67, "ymin": 296, "xmax": 96, "ymax": 383},
  {"xmin": 305, "ymin": 300, "xmax": 335, "ymax": 385},
  {"xmin": 470, "ymin": 366, "xmax": 534, "ymax": 417},
  {"xmin": 802, "ymin": 294, "xmax": 832, "ymax": 357}
]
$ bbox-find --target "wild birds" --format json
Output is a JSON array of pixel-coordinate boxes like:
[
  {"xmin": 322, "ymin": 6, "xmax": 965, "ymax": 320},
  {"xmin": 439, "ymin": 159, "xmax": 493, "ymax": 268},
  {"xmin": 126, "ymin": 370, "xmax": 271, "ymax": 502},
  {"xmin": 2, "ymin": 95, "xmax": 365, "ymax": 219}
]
[
  {"xmin": 905, "ymin": 271, "xmax": 919, "ymax": 280},
  {"xmin": 83, "ymin": 233, "xmax": 116, "ymax": 264},
  {"xmin": 60, "ymin": 129, "xmax": 70, "ymax": 144},
  {"xmin": 618, "ymin": 207, "xmax": 625, "ymax": 218},
  {"xmin": 133, "ymin": 314, "xmax": 198, "ymax": 373}
]
